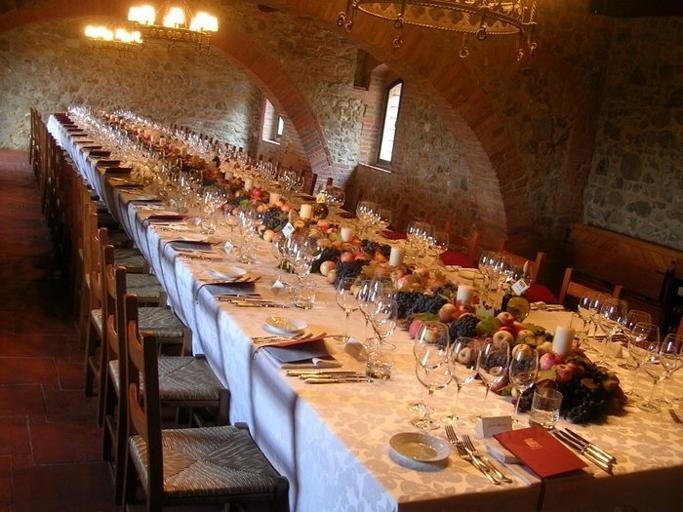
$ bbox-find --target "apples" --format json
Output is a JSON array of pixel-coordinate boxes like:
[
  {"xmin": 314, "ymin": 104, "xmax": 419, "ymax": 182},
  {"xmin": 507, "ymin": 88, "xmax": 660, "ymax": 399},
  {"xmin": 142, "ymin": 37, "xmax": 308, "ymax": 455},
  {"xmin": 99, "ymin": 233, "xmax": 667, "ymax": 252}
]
[
  {"xmin": 351, "ymin": 253, "xmax": 449, "ymax": 299},
  {"xmin": 233, "ymin": 188, "xmax": 361, "ymax": 252},
  {"xmin": 409, "ymin": 303, "xmax": 585, "ymax": 394}
]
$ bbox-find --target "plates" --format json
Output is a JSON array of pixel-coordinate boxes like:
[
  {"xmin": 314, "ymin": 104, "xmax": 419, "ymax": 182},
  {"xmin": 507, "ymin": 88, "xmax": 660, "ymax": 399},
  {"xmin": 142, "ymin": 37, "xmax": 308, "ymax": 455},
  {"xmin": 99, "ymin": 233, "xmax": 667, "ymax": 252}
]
[{"xmin": 388, "ymin": 431, "xmax": 451, "ymax": 463}]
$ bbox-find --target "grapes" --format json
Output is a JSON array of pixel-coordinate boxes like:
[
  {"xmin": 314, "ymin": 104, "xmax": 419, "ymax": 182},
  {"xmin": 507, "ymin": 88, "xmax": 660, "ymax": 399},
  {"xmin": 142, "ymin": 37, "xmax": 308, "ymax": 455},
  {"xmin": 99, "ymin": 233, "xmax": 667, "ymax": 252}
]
[
  {"xmin": 387, "ymin": 284, "xmax": 451, "ymax": 329},
  {"xmin": 512, "ymin": 368, "xmax": 628, "ymax": 423},
  {"xmin": 310, "ymin": 240, "xmax": 390, "ymax": 291}
]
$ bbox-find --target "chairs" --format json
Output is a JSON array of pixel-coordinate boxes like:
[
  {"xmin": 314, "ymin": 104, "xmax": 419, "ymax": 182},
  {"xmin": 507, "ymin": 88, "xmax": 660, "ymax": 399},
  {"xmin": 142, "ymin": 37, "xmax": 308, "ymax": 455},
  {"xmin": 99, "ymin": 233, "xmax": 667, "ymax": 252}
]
[{"xmin": 28, "ymin": 105, "xmax": 683, "ymax": 511}]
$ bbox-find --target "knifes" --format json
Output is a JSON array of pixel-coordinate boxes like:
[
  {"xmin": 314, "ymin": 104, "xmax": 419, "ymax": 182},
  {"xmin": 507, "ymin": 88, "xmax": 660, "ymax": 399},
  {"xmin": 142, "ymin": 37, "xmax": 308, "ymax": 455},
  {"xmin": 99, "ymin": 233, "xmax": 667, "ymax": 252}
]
[
  {"xmin": 558, "ymin": 429, "xmax": 611, "ymax": 465},
  {"xmin": 552, "ymin": 431, "xmax": 610, "ymax": 472},
  {"xmin": 443, "ymin": 423, "xmax": 512, "ymax": 486},
  {"xmin": 564, "ymin": 427, "xmax": 615, "ymax": 463}
]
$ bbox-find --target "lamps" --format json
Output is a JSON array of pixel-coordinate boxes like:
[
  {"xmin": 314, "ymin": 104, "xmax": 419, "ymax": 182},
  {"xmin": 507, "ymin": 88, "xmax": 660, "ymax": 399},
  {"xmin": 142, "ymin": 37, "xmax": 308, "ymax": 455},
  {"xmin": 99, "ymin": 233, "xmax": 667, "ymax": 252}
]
[
  {"xmin": 336, "ymin": 0, "xmax": 540, "ymax": 67},
  {"xmin": 84, "ymin": 0, "xmax": 219, "ymax": 52}
]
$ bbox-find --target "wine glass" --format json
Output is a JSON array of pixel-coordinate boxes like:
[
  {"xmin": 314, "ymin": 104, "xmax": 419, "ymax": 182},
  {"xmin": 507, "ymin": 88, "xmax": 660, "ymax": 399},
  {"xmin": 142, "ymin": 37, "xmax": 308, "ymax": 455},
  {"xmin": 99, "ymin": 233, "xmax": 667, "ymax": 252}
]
[{"xmin": 64, "ymin": 101, "xmax": 683, "ymax": 428}]
